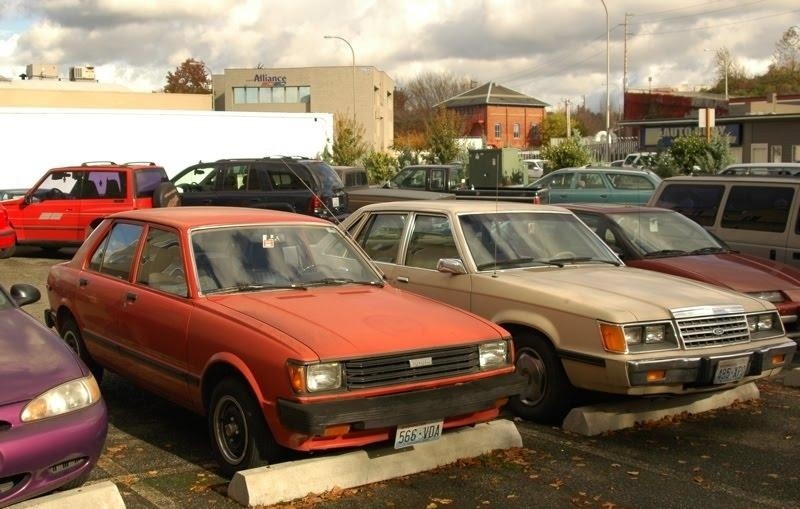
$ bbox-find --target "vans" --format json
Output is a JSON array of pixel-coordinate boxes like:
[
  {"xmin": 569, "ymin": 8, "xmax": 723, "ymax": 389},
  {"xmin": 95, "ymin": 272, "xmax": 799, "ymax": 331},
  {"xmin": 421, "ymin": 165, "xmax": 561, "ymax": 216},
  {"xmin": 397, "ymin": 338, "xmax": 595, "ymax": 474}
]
[{"xmin": 645, "ymin": 169, "xmax": 800, "ymax": 269}]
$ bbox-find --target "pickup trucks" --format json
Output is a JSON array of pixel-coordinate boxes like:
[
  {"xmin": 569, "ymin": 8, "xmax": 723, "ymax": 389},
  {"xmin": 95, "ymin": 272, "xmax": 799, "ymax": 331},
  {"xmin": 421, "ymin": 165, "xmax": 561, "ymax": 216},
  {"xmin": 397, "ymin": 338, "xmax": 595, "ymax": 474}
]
[
  {"xmin": 384, "ymin": 164, "xmax": 552, "ymax": 205},
  {"xmin": 334, "ymin": 166, "xmax": 457, "ymax": 199}
]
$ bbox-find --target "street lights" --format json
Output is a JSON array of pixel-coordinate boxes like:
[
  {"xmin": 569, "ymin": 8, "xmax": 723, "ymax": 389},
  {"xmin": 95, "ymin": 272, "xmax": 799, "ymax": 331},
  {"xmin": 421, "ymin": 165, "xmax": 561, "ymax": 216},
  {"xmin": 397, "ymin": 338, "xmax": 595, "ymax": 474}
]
[{"xmin": 322, "ymin": 34, "xmax": 356, "ymax": 153}]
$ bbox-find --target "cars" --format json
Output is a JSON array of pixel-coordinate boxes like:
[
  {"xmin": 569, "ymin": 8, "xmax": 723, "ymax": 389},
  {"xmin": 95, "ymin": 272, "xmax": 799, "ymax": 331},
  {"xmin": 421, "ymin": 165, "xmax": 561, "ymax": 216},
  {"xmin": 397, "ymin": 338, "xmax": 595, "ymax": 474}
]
[
  {"xmin": 1, "ymin": 267, "xmax": 111, "ymax": 509},
  {"xmin": 525, "ymin": 153, "xmax": 799, "ymax": 197},
  {"xmin": 555, "ymin": 193, "xmax": 798, "ymax": 350},
  {"xmin": 343, "ymin": 197, "xmax": 794, "ymax": 431},
  {"xmin": 42, "ymin": 204, "xmax": 516, "ymax": 477}
]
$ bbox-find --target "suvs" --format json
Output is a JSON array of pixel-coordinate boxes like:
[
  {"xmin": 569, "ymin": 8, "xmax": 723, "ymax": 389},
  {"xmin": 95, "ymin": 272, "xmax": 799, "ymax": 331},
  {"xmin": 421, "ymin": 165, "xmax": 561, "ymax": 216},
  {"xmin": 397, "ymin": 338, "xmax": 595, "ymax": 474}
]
[
  {"xmin": 0, "ymin": 159, "xmax": 169, "ymax": 262},
  {"xmin": 163, "ymin": 155, "xmax": 352, "ymax": 218}
]
